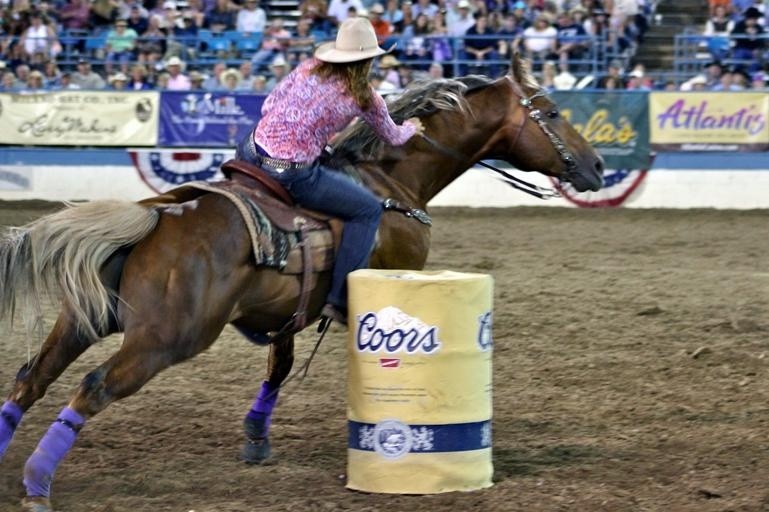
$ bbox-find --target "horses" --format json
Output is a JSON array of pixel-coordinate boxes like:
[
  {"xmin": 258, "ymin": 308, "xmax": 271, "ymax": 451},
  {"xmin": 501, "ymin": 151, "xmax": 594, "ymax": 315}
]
[{"xmin": 0, "ymin": 52, "xmax": 606, "ymax": 512}]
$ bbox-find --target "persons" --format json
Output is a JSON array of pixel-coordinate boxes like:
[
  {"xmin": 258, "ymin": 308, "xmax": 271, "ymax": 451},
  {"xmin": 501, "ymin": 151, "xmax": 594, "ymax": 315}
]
[
  {"xmin": 1, "ymin": 1, "xmax": 367, "ymax": 93},
  {"xmin": 236, "ymin": 16, "xmax": 425, "ymax": 326},
  {"xmin": 681, "ymin": 1, "xmax": 764, "ymax": 92},
  {"xmin": 364, "ymin": 1, "xmax": 654, "ymax": 92}
]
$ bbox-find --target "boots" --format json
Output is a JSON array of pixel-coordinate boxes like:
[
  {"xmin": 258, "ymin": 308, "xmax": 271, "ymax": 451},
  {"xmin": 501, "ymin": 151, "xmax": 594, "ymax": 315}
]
[{"xmin": 320, "ymin": 303, "xmax": 347, "ymax": 325}]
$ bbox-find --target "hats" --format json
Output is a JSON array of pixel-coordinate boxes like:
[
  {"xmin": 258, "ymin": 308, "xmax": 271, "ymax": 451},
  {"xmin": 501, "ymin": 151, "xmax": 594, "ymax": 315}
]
[
  {"xmin": 741, "ymin": 6, "xmax": 764, "ymax": 18},
  {"xmin": 0, "ymin": 55, "xmax": 286, "ymax": 91},
  {"xmin": 457, "ymin": 0, "xmax": 638, "ymax": 26},
  {"xmin": 377, "ymin": 54, "xmax": 400, "ymax": 69},
  {"xmin": 314, "ymin": 17, "xmax": 397, "ymax": 64},
  {"xmin": 598, "ymin": 60, "xmax": 769, "ymax": 89}
]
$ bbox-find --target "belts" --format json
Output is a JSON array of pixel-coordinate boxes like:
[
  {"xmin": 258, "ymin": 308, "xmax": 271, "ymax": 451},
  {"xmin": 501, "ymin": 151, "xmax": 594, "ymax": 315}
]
[{"xmin": 247, "ymin": 128, "xmax": 313, "ymax": 169}]
